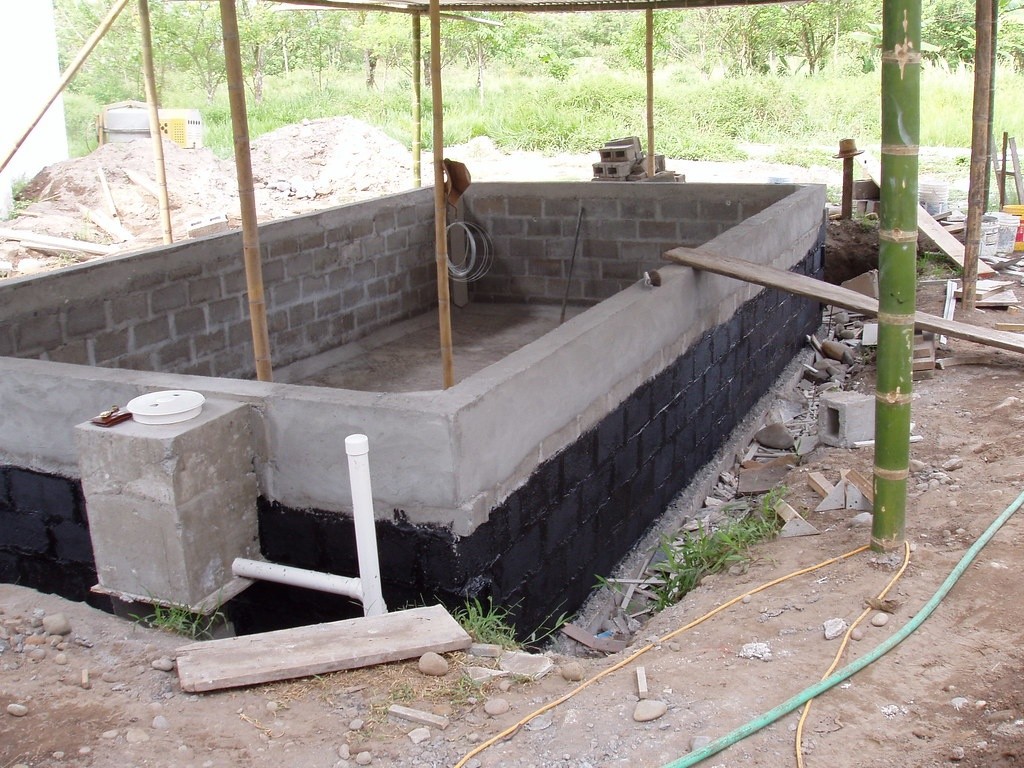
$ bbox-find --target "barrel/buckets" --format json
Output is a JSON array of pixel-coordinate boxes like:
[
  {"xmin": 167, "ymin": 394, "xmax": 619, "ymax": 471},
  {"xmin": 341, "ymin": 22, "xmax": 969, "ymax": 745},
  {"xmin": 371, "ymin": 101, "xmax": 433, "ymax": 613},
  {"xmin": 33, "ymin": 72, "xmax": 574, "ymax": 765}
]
[
  {"xmin": 1003, "ymin": 205, "xmax": 1024, "ymax": 252},
  {"xmin": 918, "ymin": 182, "xmax": 946, "ymax": 216},
  {"xmin": 963, "ymin": 215, "xmax": 998, "ymax": 257},
  {"xmin": 996, "ymin": 216, "xmax": 1021, "ymax": 253},
  {"xmin": 985, "ymin": 211, "xmax": 1012, "ymax": 222}
]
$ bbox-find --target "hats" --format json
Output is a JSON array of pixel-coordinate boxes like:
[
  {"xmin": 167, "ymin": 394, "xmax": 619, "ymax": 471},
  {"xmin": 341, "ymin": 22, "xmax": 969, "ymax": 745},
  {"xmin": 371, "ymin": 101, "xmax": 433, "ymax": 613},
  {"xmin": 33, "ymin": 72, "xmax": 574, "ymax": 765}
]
[{"xmin": 443, "ymin": 158, "xmax": 471, "ymax": 204}]
